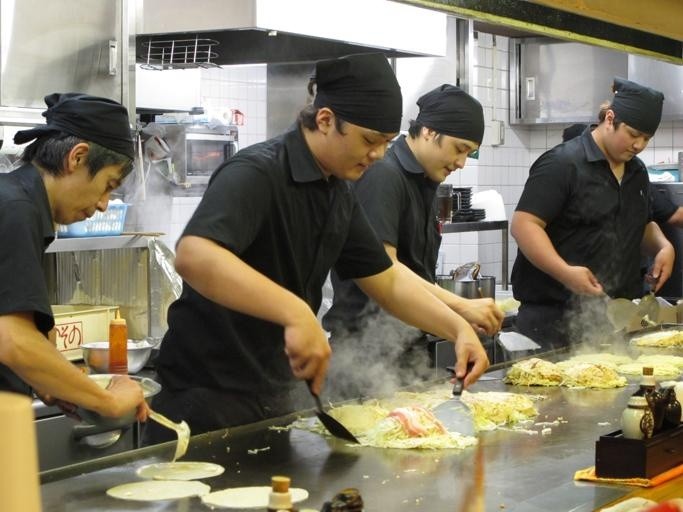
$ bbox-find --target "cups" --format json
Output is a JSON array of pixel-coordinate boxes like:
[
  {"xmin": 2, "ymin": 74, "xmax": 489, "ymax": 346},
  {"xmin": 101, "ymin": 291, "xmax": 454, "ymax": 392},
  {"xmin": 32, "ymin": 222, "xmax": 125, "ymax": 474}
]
[{"xmin": 435, "ymin": 183, "xmax": 462, "ymax": 224}]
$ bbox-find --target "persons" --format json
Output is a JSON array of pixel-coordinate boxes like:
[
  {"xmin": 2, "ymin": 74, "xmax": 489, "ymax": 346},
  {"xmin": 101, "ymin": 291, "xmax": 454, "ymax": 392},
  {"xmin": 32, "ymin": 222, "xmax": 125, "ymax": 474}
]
[
  {"xmin": 0, "ymin": 90, "xmax": 148, "ymax": 511},
  {"xmin": 564, "ymin": 122, "xmax": 683, "ymax": 230},
  {"xmin": 321, "ymin": 83, "xmax": 504, "ymax": 399},
  {"xmin": 511, "ymin": 77, "xmax": 678, "ymax": 351},
  {"xmin": 140, "ymin": 52, "xmax": 491, "ymax": 444}
]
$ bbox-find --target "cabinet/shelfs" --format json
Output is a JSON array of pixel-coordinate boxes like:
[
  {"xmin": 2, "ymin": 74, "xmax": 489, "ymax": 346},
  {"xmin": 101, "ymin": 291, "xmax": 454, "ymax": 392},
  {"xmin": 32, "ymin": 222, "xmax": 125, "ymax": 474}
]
[
  {"xmin": 0, "ymin": 0, "xmax": 142, "ymax": 132},
  {"xmin": 504, "ymin": 26, "xmax": 683, "ymax": 123}
]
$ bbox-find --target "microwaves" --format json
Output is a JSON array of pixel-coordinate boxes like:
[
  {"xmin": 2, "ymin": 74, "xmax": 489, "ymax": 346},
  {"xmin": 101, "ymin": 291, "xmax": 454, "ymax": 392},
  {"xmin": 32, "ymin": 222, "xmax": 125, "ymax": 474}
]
[{"xmin": 159, "ymin": 122, "xmax": 240, "ymax": 196}]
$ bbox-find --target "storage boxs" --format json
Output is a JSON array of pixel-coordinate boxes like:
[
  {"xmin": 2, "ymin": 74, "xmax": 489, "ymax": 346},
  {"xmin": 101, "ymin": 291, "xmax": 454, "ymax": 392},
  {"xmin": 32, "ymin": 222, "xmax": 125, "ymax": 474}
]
[{"xmin": 46, "ymin": 303, "xmax": 121, "ymax": 362}]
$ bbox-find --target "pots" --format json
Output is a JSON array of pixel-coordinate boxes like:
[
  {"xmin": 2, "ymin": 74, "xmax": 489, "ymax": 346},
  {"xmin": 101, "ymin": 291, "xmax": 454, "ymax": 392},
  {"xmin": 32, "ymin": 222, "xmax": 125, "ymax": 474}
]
[{"xmin": 437, "ymin": 274, "xmax": 496, "ymax": 304}]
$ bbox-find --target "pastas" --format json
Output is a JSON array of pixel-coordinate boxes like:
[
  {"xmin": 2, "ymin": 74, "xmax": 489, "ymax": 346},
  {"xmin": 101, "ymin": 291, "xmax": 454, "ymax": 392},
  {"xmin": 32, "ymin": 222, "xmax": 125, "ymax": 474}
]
[{"xmin": 316, "ymin": 398, "xmax": 447, "ymax": 442}]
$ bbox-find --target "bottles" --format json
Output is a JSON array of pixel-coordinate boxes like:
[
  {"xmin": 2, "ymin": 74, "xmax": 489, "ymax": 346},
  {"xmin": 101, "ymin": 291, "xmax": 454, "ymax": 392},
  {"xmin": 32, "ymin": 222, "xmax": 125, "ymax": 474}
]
[
  {"xmin": 266, "ymin": 476, "xmax": 294, "ymax": 512},
  {"xmin": 621, "ymin": 365, "xmax": 682, "ymax": 440},
  {"xmin": 109, "ymin": 308, "xmax": 129, "ymax": 375}
]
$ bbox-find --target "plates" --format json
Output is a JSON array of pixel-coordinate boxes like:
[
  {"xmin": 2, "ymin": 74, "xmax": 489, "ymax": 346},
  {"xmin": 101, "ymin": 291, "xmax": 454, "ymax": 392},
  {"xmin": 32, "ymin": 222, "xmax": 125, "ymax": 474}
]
[{"xmin": 452, "ymin": 186, "xmax": 486, "ymax": 222}]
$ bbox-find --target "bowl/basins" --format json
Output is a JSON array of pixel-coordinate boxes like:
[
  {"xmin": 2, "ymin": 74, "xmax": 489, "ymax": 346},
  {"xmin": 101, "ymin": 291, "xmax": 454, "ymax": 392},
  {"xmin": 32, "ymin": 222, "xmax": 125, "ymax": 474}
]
[
  {"xmin": 73, "ymin": 374, "xmax": 162, "ymax": 428},
  {"xmin": 78, "ymin": 340, "xmax": 154, "ymax": 376},
  {"xmin": 143, "ymin": 337, "xmax": 163, "ymax": 369}
]
